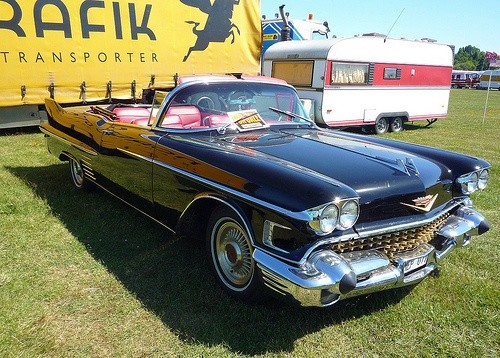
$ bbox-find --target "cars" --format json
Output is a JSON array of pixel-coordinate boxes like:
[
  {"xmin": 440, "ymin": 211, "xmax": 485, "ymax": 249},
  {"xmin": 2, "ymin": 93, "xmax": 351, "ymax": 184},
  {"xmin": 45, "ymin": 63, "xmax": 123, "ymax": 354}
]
[{"xmin": 30, "ymin": 69, "xmax": 492, "ymax": 312}]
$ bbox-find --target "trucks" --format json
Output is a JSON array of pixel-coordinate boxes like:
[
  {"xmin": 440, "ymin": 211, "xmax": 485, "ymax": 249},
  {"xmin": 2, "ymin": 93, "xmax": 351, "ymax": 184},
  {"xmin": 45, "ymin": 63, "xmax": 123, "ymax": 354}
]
[
  {"xmin": 478, "ymin": 70, "xmax": 500, "ymax": 89},
  {"xmin": 262, "ymin": 32, "xmax": 455, "ymax": 136}
]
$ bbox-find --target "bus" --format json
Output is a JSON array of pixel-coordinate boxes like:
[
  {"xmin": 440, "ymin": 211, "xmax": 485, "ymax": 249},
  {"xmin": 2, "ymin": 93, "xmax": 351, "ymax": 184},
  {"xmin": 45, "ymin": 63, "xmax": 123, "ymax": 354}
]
[{"xmin": 451, "ymin": 71, "xmax": 480, "ymax": 89}]
